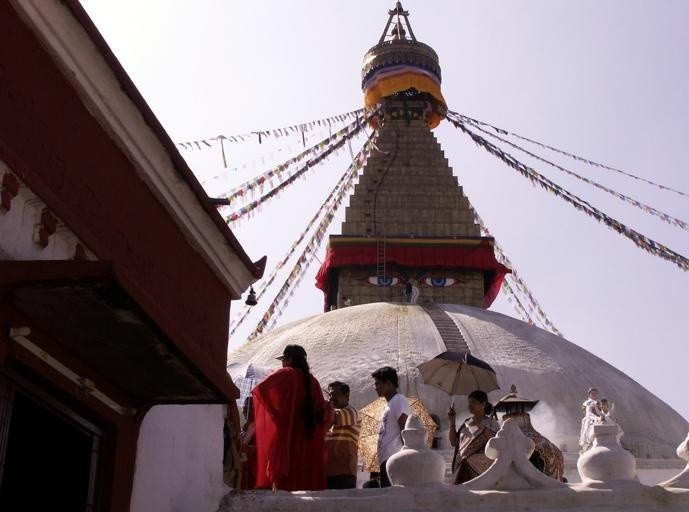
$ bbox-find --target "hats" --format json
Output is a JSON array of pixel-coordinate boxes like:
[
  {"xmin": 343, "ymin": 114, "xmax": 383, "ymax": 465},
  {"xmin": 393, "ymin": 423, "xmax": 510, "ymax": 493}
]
[
  {"xmin": 275, "ymin": 345, "xmax": 307, "ymax": 360},
  {"xmin": 588, "ymin": 388, "xmax": 598, "ymax": 394}
]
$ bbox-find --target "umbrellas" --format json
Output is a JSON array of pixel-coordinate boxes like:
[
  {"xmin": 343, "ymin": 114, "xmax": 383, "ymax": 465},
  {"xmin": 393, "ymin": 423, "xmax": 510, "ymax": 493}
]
[
  {"xmin": 417, "ymin": 351, "xmax": 500, "ymax": 410},
  {"xmin": 226, "ymin": 362, "xmax": 275, "ymax": 421},
  {"xmin": 357, "ymin": 396, "xmax": 440, "ymax": 472}
]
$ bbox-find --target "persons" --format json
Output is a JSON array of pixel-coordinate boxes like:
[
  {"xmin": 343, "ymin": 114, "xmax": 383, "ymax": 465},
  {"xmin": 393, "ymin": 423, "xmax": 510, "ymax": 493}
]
[
  {"xmin": 363, "ymin": 472, "xmax": 380, "ymax": 488},
  {"xmin": 243, "ymin": 397, "xmax": 256, "ymax": 456},
  {"xmin": 325, "ymin": 381, "xmax": 358, "ymax": 489},
  {"xmin": 448, "ymin": 390, "xmax": 501, "ymax": 484},
  {"xmin": 580, "ymin": 387, "xmax": 610, "ymax": 435},
  {"xmin": 251, "ymin": 344, "xmax": 336, "ymax": 491},
  {"xmin": 371, "ymin": 366, "xmax": 412, "ymax": 488}
]
